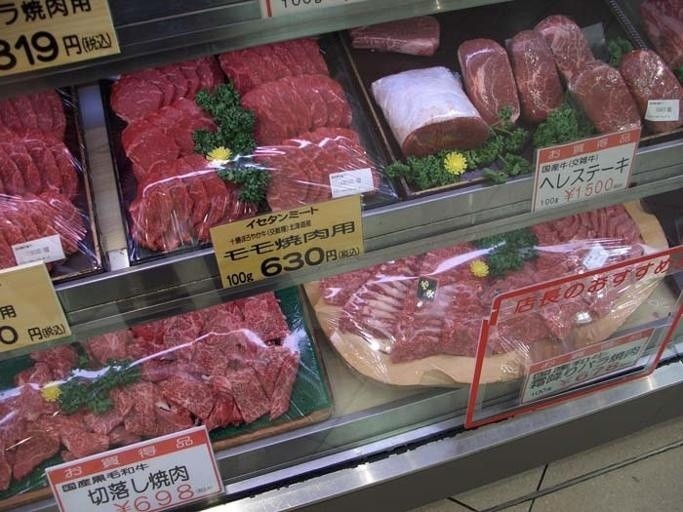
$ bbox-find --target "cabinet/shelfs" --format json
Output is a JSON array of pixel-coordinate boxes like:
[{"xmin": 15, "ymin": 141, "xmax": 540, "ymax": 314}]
[{"xmin": 0, "ymin": 0, "xmax": 683, "ymax": 512}]
